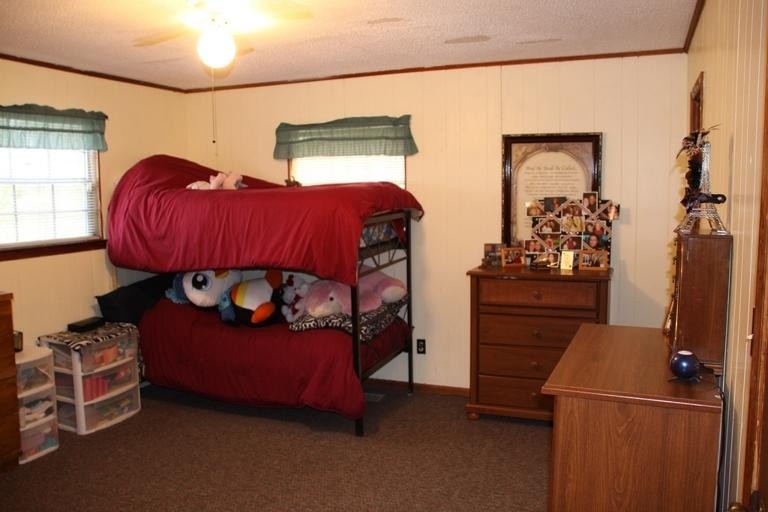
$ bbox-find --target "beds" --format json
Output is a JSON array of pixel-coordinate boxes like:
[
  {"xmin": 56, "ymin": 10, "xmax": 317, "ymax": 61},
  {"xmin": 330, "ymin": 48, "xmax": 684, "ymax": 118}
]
[{"xmin": 96, "ymin": 154, "xmax": 416, "ymax": 438}]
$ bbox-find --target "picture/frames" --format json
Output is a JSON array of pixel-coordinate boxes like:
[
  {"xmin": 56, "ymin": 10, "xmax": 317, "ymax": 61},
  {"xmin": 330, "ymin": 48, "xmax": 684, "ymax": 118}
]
[{"xmin": 502, "ymin": 132, "xmax": 602, "ymax": 247}]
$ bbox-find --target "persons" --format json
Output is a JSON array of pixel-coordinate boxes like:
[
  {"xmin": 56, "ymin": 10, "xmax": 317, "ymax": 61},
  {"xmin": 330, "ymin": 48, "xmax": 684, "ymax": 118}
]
[
  {"xmin": 583, "ymin": 197, "xmax": 589, "ymax": 208},
  {"xmin": 545, "ymin": 234, "xmax": 553, "ymax": 251},
  {"xmin": 593, "ymin": 222, "xmax": 603, "ymax": 234},
  {"xmin": 563, "ymin": 240, "xmax": 568, "ymax": 249},
  {"xmin": 514, "ymin": 253, "xmax": 522, "ymax": 264},
  {"xmin": 584, "ymin": 223, "xmax": 594, "ymax": 234},
  {"xmin": 550, "ymin": 221, "xmax": 559, "ymax": 232},
  {"xmin": 535, "ymin": 240, "xmax": 545, "ymax": 252},
  {"xmin": 587, "ymin": 195, "xmax": 596, "ymax": 213},
  {"xmin": 505, "ymin": 255, "xmax": 513, "ymax": 264},
  {"xmin": 588, "ymin": 235, "xmax": 602, "ymax": 250},
  {"xmin": 568, "ymin": 206, "xmax": 574, "ymax": 216},
  {"xmin": 548, "ymin": 253, "xmax": 557, "ymax": 267},
  {"xmin": 526, "ymin": 241, "xmax": 535, "ymax": 252},
  {"xmin": 541, "ymin": 221, "xmax": 552, "ymax": 232},
  {"xmin": 572, "ymin": 206, "xmax": 579, "ymax": 216},
  {"xmin": 567, "ymin": 238, "xmax": 576, "ymax": 249},
  {"xmin": 590, "ymin": 256, "xmax": 600, "ymax": 267}
]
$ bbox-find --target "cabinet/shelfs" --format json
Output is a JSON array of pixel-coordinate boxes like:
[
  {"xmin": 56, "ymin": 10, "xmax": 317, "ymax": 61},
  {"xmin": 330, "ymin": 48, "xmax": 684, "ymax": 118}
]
[
  {"xmin": 465, "ymin": 264, "xmax": 614, "ymax": 421},
  {"xmin": 42, "ymin": 331, "xmax": 142, "ymax": 435},
  {"xmin": 540, "ymin": 323, "xmax": 724, "ymax": 512},
  {"xmin": 14, "ymin": 345, "xmax": 58, "ymax": 464}
]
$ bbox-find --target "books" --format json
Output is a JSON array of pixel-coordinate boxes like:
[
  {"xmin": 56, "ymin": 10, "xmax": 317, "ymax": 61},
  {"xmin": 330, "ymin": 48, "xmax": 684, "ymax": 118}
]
[
  {"xmin": 50, "ymin": 345, "xmax": 72, "ymax": 369},
  {"xmin": 58, "ymin": 378, "xmax": 108, "ymax": 402}
]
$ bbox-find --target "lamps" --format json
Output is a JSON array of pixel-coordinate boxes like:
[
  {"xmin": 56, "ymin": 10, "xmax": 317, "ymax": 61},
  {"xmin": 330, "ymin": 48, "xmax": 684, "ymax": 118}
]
[{"xmin": 177, "ymin": 2, "xmax": 277, "ymax": 70}]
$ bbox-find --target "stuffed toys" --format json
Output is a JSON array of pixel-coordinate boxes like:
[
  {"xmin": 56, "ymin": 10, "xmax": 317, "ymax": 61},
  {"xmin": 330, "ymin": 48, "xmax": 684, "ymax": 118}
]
[
  {"xmin": 166, "ymin": 268, "xmax": 283, "ymax": 326},
  {"xmin": 282, "ymin": 274, "xmax": 311, "ymax": 323},
  {"xmin": 185, "ymin": 171, "xmax": 249, "ymax": 190},
  {"xmin": 306, "ymin": 264, "xmax": 406, "ymax": 317}
]
